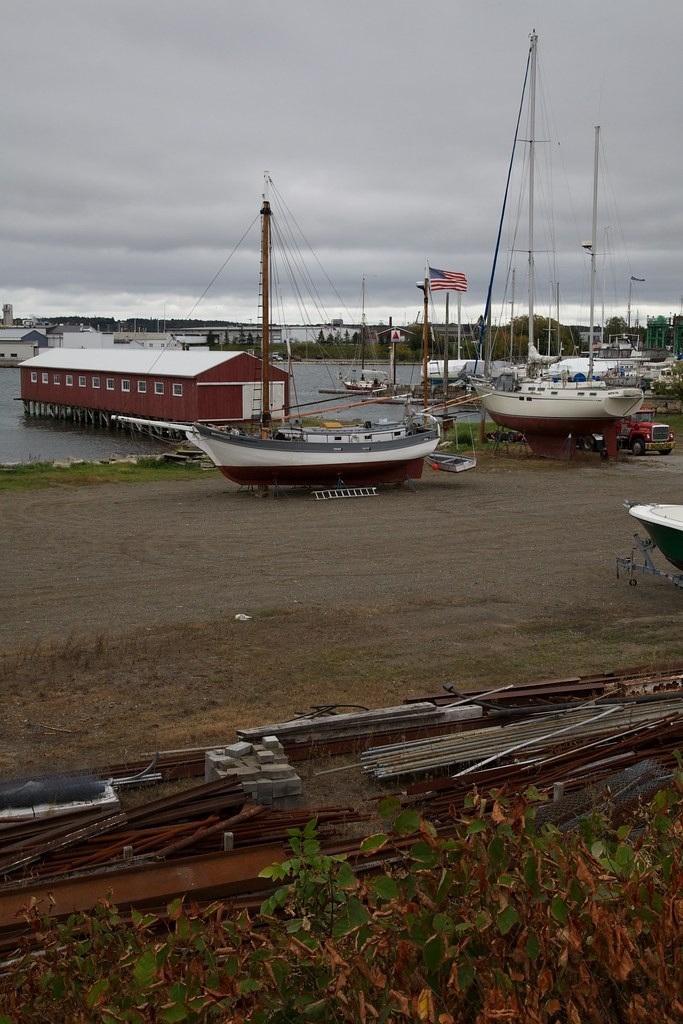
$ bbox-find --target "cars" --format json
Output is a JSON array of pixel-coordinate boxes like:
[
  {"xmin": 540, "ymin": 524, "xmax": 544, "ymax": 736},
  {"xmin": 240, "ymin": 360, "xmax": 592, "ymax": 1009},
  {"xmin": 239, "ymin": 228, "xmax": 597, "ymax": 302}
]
[{"xmin": 268, "ymin": 350, "xmax": 322, "ymax": 362}]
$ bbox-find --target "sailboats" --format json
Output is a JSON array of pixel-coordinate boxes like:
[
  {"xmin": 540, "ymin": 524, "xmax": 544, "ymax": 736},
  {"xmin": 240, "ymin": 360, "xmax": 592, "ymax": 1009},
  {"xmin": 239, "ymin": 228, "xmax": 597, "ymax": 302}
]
[
  {"xmin": 183, "ymin": 170, "xmax": 443, "ymax": 496},
  {"xmin": 472, "ymin": 32, "xmax": 650, "ymax": 463},
  {"xmin": 338, "ymin": 124, "xmax": 662, "ymax": 401}
]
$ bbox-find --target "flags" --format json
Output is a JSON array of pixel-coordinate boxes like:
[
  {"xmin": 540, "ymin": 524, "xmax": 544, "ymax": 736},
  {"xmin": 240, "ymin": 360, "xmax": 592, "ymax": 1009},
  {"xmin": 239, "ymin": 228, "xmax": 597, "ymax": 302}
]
[
  {"xmin": 428, "ymin": 266, "xmax": 468, "ymax": 293},
  {"xmin": 592, "ymin": 341, "xmax": 601, "ymax": 349}
]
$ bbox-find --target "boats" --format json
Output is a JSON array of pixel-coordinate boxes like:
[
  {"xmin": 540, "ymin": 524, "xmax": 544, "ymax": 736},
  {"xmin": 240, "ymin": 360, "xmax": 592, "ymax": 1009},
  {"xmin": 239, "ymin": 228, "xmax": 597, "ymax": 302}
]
[
  {"xmin": 426, "ymin": 419, "xmax": 479, "ymax": 472},
  {"xmin": 616, "ymin": 499, "xmax": 683, "ymax": 592}
]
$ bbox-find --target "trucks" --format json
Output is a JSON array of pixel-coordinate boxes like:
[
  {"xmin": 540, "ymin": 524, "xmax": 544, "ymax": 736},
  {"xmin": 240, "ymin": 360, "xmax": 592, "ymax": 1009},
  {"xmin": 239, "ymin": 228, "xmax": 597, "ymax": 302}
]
[{"xmin": 573, "ymin": 408, "xmax": 675, "ymax": 455}]
